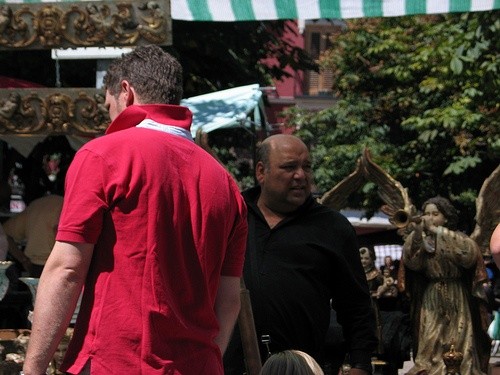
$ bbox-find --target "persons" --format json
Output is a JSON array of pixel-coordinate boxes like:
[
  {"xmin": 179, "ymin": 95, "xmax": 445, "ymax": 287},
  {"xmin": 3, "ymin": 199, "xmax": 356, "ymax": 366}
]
[
  {"xmin": 482, "ymin": 223, "xmax": 500, "ymax": 357},
  {"xmin": 24, "ymin": 152, "xmax": 65, "ymax": 201},
  {"xmin": 223, "ymin": 134, "xmax": 378, "ymax": 375},
  {"xmin": 260, "ymin": 350, "xmax": 325, "ymax": 375},
  {"xmin": 3, "ymin": 183, "xmax": 64, "ymax": 278},
  {"xmin": 359, "ymin": 246, "xmax": 411, "ymax": 375},
  {"xmin": 403, "ymin": 197, "xmax": 489, "ymax": 375},
  {"xmin": 19, "ymin": 44, "xmax": 248, "ymax": 375}
]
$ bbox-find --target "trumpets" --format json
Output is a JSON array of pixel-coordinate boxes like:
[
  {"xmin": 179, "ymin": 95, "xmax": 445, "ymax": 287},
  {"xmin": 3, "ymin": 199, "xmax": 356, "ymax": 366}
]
[{"xmin": 391, "ymin": 210, "xmax": 422, "ymax": 228}]
